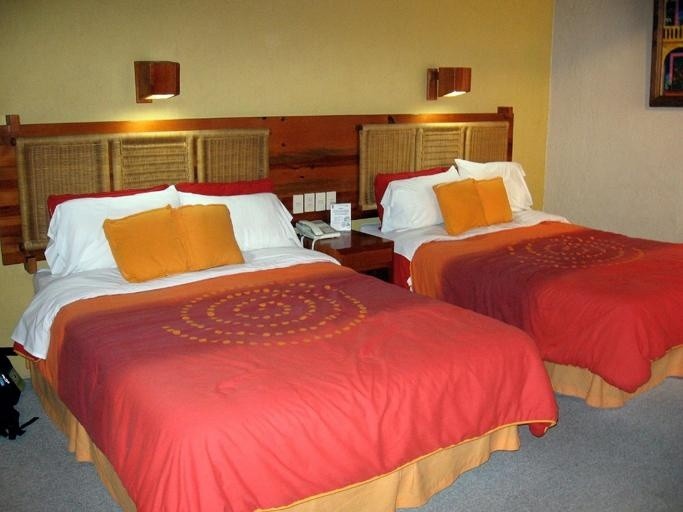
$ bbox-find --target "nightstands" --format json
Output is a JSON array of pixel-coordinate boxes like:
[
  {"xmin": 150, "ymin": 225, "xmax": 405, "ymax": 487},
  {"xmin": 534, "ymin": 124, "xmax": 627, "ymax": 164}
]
[{"xmin": 292, "ymin": 227, "xmax": 397, "ymax": 290}]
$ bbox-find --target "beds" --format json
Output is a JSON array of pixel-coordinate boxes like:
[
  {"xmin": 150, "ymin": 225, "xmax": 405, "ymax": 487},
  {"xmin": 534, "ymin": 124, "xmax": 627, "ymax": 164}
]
[
  {"xmin": 10, "ymin": 213, "xmax": 561, "ymax": 511},
  {"xmin": 356, "ymin": 203, "xmax": 683, "ymax": 413}
]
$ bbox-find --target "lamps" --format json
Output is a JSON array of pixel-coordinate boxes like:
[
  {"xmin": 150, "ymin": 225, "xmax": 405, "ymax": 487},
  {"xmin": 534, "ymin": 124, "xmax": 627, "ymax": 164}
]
[
  {"xmin": 422, "ymin": 65, "xmax": 474, "ymax": 103},
  {"xmin": 130, "ymin": 55, "xmax": 185, "ymax": 105}
]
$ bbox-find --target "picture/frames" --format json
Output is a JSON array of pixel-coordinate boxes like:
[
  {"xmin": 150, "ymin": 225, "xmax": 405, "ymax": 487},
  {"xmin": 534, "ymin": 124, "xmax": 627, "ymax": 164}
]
[{"xmin": 647, "ymin": 0, "xmax": 683, "ymax": 108}]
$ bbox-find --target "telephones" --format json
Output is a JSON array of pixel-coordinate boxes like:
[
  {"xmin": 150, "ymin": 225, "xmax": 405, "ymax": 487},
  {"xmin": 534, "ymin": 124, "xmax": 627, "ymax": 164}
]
[{"xmin": 296, "ymin": 219, "xmax": 341, "ymax": 240}]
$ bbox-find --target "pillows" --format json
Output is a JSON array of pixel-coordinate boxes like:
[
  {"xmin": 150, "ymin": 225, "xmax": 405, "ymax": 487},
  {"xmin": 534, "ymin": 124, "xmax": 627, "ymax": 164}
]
[
  {"xmin": 452, "ymin": 155, "xmax": 533, "ymax": 215},
  {"xmin": 174, "ymin": 176, "xmax": 276, "ymax": 197},
  {"xmin": 367, "ymin": 163, "xmax": 451, "ymax": 233},
  {"xmin": 98, "ymin": 200, "xmax": 192, "ymax": 281},
  {"xmin": 430, "ymin": 174, "xmax": 488, "ymax": 234},
  {"xmin": 44, "ymin": 183, "xmax": 170, "ymax": 222},
  {"xmin": 41, "ymin": 182, "xmax": 185, "ymax": 279},
  {"xmin": 177, "ymin": 185, "xmax": 305, "ymax": 254},
  {"xmin": 374, "ymin": 163, "xmax": 460, "ymax": 234},
  {"xmin": 475, "ymin": 172, "xmax": 514, "ymax": 228},
  {"xmin": 174, "ymin": 199, "xmax": 246, "ymax": 273}
]
